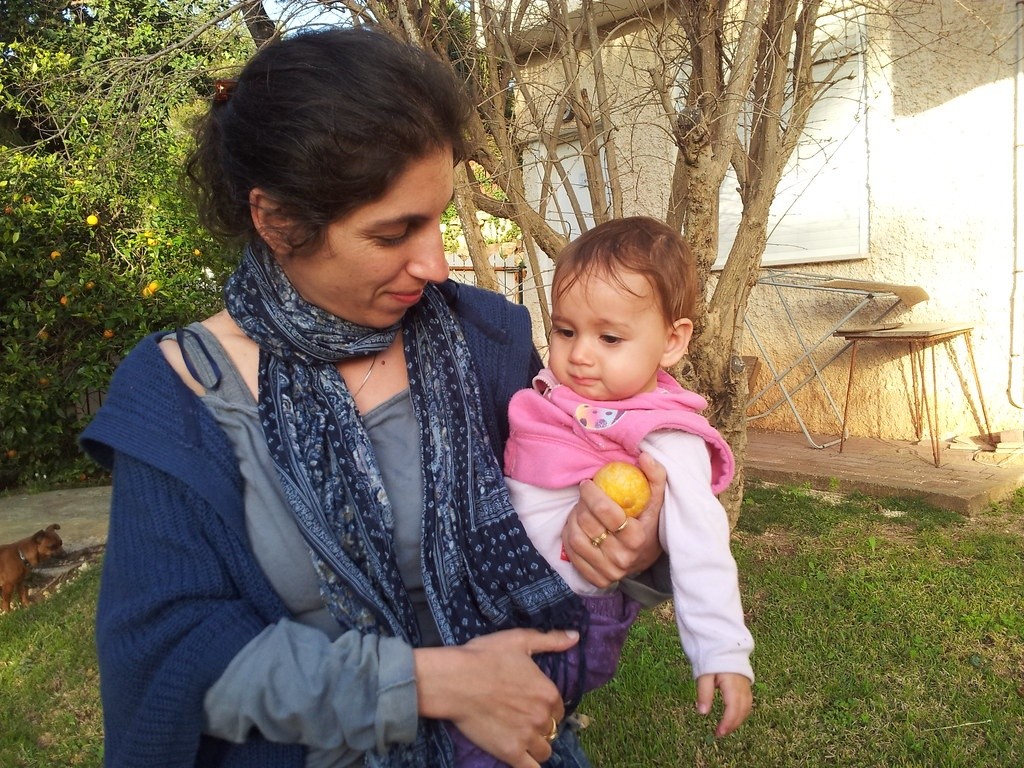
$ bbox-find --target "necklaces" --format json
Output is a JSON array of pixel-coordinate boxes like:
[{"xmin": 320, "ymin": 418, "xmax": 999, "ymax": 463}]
[{"xmin": 338, "ymin": 353, "xmax": 378, "ymax": 404}]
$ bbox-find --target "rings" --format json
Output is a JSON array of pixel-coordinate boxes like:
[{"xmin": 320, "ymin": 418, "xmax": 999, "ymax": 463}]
[
  {"xmin": 543, "ymin": 718, "xmax": 559, "ymax": 746},
  {"xmin": 607, "ymin": 515, "xmax": 630, "ymax": 535},
  {"xmin": 591, "ymin": 528, "xmax": 612, "ymax": 549}
]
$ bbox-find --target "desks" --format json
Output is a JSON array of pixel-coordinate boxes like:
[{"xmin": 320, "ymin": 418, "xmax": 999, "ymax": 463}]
[{"xmin": 833, "ymin": 323, "xmax": 993, "ymax": 467}]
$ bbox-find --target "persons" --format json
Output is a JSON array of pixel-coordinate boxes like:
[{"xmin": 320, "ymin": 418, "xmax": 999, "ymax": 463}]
[
  {"xmin": 458, "ymin": 217, "xmax": 756, "ymax": 768},
  {"xmin": 76, "ymin": 29, "xmax": 682, "ymax": 768}
]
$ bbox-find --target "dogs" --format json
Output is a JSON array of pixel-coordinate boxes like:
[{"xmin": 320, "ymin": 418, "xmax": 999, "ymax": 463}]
[{"xmin": 0, "ymin": 523, "xmax": 68, "ymax": 614}]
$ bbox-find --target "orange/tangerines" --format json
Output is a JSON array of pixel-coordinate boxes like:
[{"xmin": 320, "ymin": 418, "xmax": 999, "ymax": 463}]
[
  {"xmin": 3, "ymin": 194, "xmax": 201, "ymax": 461},
  {"xmin": 592, "ymin": 461, "xmax": 651, "ymax": 518}
]
